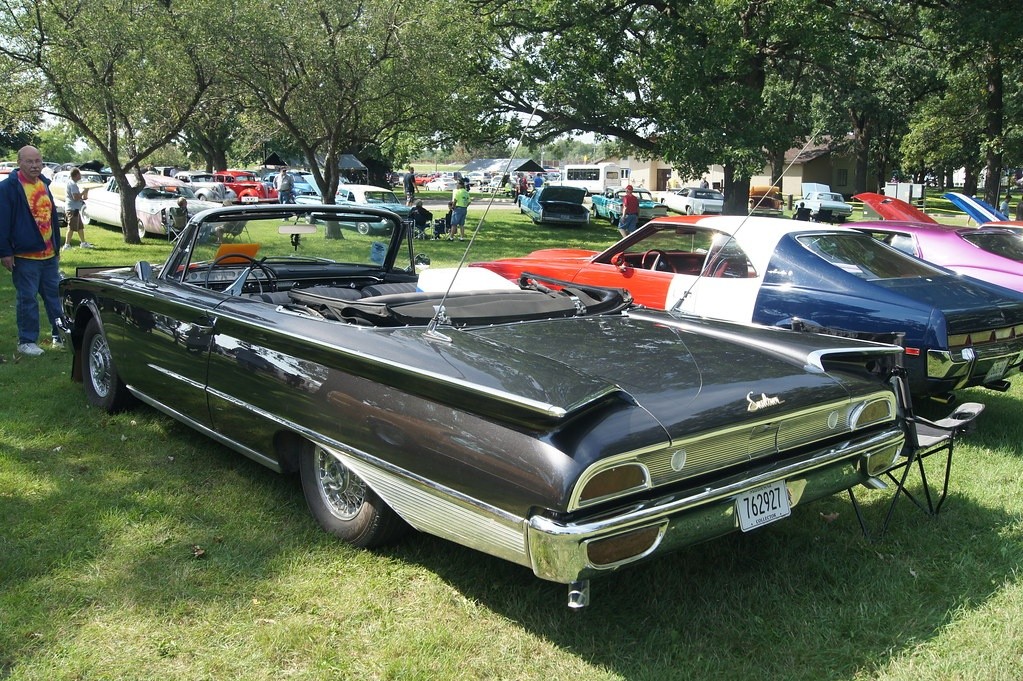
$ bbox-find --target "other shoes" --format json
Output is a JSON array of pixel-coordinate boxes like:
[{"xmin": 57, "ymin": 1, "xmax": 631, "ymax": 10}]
[
  {"xmin": 459, "ymin": 238, "xmax": 464, "ymax": 241},
  {"xmin": 447, "ymin": 238, "xmax": 453, "ymax": 241}
]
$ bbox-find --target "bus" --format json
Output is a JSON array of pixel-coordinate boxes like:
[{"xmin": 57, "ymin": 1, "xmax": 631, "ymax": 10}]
[{"xmin": 561, "ymin": 161, "xmax": 622, "ymax": 195}]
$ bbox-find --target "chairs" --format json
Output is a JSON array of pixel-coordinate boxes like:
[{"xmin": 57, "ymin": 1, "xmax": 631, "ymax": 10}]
[
  {"xmin": 796, "ymin": 208, "xmax": 833, "ymax": 222},
  {"xmin": 167, "ymin": 208, "xmax": 253, "ymax": 245},
  {"xmin": 705, "ymin": 245, "xmax": 749, "ymax": 276},
  {"xmin": 411, "ymin": 215, "xmax": 453, "ymax": 241},
  {"xmin": 249, "ymin": 282, "xmax": 418, "ymax": 306},
  {"xmin": 848, "ymin": 365, "xmax": 985, "ymax": 540}
]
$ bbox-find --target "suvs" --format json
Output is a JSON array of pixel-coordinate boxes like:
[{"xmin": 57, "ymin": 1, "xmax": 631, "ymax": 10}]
[
  {"xmin": 211, "ymin": 171, "xmax": 281, "ymax": 205},
  {"xmin": 173, "ymin": 170, "xmax": 238, "ymax": 205}
]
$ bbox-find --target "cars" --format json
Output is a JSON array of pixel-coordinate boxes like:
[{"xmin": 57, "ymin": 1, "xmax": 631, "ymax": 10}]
[
  {"xmin": 425, "ymin": 178, "xmax": 460, "ymax": 192},
  {"xmin": 656, "ymin": 187, "xmax": 724, "ymax": 216},
  {"xmin": 517, "ymin": 184, "xmax": 590, "ymax": 225},
  {"xmin": 849, "ymin": 190, "xmax": 1013, "ymax": 227},
  {"xmin": 833, "ymin": 221, "xmax": 1023, "ymax": 295},
  {"xmin": 459, "ymin": 212, "xmax": 1023, "ymax": 429},
  {"xmin": 78, "ymin": 174, "xmax": 237, "ymax": 240},
  {"xmin": 0, "ymin": 161, "xmax": 289, "ymax": 185},
  {"xmin": 794, "ymin": 191, "xmax": 854, "ymax": 223},
  {"xmin": 747, "ymin": 185, "xmax": 785, "ymax": 216},
  {"xmin": 398, "ymin": 171, "xmax": 561, "ymax": 192},
  {"xmin": 294, "ymin": 174, "xmax": 415, "ymax": 237},
  {"xmin": 56, "ymin": 203, "xmax": 905, "ymax": 608}
]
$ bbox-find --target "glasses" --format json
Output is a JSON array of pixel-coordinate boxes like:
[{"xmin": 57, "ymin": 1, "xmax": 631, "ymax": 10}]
[{"xmin": 19, "ymin": 158, "xmax": 43, "ymax": 165}]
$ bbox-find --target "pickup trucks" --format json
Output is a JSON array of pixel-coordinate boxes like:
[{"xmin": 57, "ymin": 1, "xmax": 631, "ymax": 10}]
[{"xmin": 591, "ymin": 186, "xmax": 669, "ymax": 227}]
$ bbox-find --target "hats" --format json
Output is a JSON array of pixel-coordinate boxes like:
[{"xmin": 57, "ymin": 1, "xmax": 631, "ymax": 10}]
[{"xmin": 626, "ymin": 185, "xmax": 633, "ymax": 190}]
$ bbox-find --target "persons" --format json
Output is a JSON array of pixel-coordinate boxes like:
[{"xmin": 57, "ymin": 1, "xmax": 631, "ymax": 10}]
[
  {"xmin": 699, "ymin": 176, "xmax": 709, "ymax": 189},
  {"xmin": 403, "ymin": 167, "xmax": 419, "ymax": 207},
  {"xmin": 141, "ymin": 164, "xmax": 157, "ymax": 174},
  {"xmin": 215, "ymin": 199, "xmax": 238, "ymax": 243},
  {"xmin": 1015, "ymin": 196, "xmax": 1023, "ymax": 221},
  {"xmin": 794, "ymin": 202, "xmax": 805, "ymax": 220},
  {"xmin": 445, "ymin": 172, "xmax": 471, "ymax": 241},
  {"xmin": 177, "ymin": 197, "xmax": 194, "ymax": 223},
  {"xmin": 273, "ymin": 167, "xmax": 295, "ymax": 204},
  {"xmin": 62, "ymin": 168, "xmax": 94, "ymax": 249},
  {"xmin": 0, "ymin": 146, "xmax": 66, "ymax": 354},
  {"xmin": 616, "ymin": 185, "xmax": 640, "ymax": 237},
  {"xmin": 505, "ymin": 172, "xmax": 544, "ymax": 196},
  {"xmin": 408, "ymin": 198, "xmax": 432, "ymax": 227},
  {"xmin": 169, "ymin": 165, "xmax": 179, "ymax": 177},
  {"xmin": 999, "ymin": 194, "xmax": 1015, "ymax": 218}
]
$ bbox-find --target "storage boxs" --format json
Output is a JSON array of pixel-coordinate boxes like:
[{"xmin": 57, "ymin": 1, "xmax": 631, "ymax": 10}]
[{"xmin": 417, "ymin": 268, "xmax": 522, "ymax": 294}]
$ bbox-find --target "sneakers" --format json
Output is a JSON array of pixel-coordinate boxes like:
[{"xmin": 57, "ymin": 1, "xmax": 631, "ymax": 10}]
[
  {"xmin": 17, "ymin": 343, "xmax": 45, "ymax": 356},
  {"xmin": 79, "ymin": 241, "xmax": 95, "ymax": 250},
  {"xmin": 51, "ymin": 337, "xmax": 65, "ymax": 350},
  {"xmin": 61, "ymin": 244, "xmax": 77, "ymax": 252}
]
